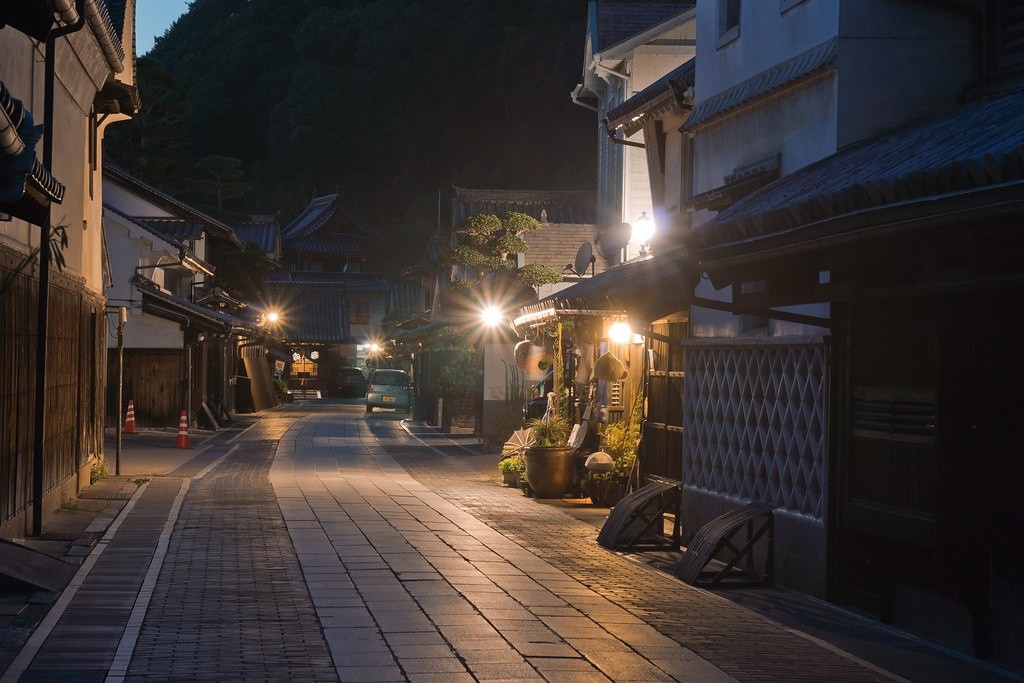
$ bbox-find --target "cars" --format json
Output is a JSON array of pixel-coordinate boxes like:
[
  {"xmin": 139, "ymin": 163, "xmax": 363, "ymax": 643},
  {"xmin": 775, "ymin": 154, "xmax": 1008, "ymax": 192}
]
[{"xmin": 336, "ymin": 366, "xmax": 366, "ymax": 394}]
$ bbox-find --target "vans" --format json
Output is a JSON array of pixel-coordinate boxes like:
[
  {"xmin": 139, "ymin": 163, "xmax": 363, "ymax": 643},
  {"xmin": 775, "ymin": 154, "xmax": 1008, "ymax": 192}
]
[{"xmin": 366, "ymin": 369, "xmax": 412, "ymax": 414}]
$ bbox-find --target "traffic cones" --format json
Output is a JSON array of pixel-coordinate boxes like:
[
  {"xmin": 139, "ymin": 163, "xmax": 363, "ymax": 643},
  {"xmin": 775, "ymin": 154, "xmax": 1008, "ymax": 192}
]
[
  {"xmin": 176, "ymin": 409, "xmax": 190, "ymax": 449},
  {"xmin": 121, "ymin": 400, "xmax": 139, "ymax": 434}
]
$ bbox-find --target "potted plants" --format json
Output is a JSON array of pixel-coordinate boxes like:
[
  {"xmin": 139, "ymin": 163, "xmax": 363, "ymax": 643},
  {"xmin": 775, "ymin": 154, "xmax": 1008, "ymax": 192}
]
[{"xmin": 499, "ymin": 415, "xmax": 640, "ymax": 507}]
